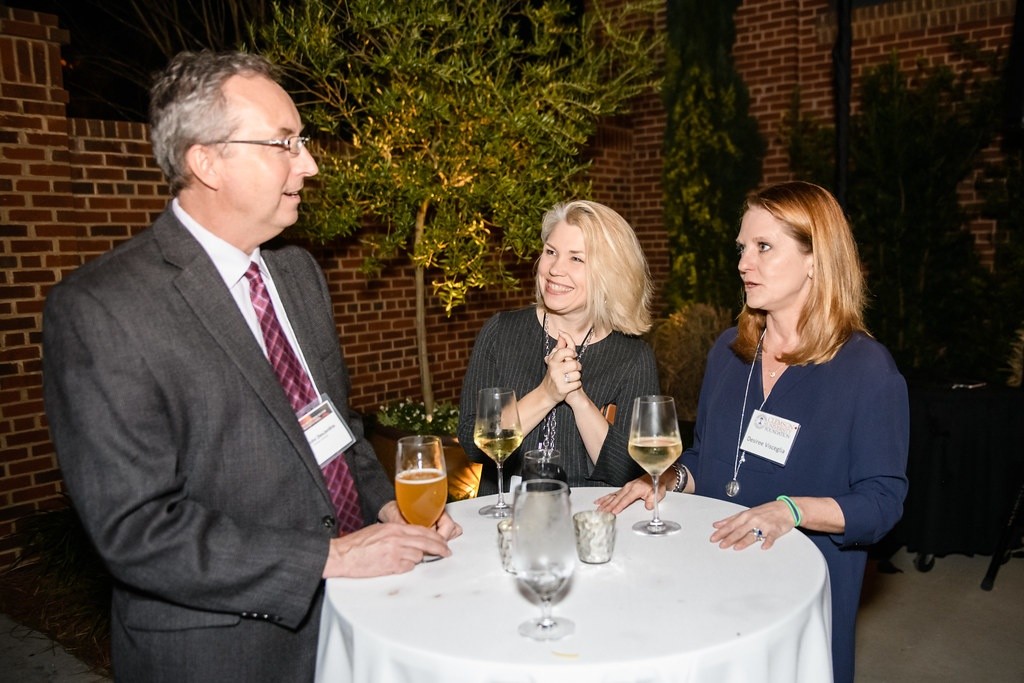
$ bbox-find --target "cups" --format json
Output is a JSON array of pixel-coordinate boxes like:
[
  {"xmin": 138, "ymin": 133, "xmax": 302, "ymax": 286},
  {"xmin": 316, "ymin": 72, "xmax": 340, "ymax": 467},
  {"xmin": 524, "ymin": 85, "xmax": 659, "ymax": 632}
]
[
  {"xmin": 522, "ymin": 448, "xmax": 570, "ymax": 501},
  {"xmin": 573, "ymin": 509, "xmax": 616, "ymax": 564},
  {"xmin": 497, "ymin": 517, "xmax": 519, "ymax": 575}
]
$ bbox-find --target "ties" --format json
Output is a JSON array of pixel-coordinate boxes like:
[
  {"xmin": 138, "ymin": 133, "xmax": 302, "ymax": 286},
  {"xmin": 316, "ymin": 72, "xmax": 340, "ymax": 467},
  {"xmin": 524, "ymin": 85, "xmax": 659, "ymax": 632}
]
[{"xmin": 244, "ymin": 261, "xmax": 364, "ymax": 539}]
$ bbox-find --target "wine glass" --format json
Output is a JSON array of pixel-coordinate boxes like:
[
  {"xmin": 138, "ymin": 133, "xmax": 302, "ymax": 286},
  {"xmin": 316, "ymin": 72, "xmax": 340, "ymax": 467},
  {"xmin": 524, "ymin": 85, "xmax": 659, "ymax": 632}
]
[
  {"xmin": 473, "ymin": 388, "xmax": 524, "ymax": 519},
  {"xmin": 394, "ymin": 435, "xmax": 449, "ymax": 563},
  {"xmin": 628, "ymin": 395, "xmax": 683, "ymax": 537},
  {"xmin": 510, "ymin": 478, "xmax": 578, "ymax": 642}
]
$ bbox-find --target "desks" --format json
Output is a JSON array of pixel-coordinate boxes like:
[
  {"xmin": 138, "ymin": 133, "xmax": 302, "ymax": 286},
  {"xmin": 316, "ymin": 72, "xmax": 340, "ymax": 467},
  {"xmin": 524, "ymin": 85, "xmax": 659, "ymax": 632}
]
[
  {"xmin": 898, "ymin": 369, "xmax": 1024, "ymax": 562},
  {"xmin": 314, "ymin": 487, "xmax": 834, "ymax": 683}
]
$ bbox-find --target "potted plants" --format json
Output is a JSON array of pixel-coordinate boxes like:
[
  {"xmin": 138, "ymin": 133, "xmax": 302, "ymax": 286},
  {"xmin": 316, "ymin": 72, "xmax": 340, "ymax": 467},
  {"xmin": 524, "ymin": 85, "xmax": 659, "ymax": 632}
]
[{"xmin": 233, "ymin": 0, "xmax": 671, "ymax": 485}]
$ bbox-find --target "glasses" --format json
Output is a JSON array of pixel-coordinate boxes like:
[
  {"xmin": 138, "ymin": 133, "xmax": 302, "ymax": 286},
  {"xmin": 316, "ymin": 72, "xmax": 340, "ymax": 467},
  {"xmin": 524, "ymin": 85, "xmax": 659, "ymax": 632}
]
[{"xmin": 202, "ymin": 136, "xmax": 311, "ymax": 154}]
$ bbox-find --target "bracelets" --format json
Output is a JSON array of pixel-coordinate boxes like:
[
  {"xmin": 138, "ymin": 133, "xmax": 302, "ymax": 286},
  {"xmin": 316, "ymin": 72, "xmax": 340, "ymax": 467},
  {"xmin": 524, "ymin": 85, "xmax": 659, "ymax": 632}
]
[
  {"xmin": 669, "ymin": 462, "xmax": 689, "ymax": 492},
  {"xmin": 775, "ymin": 494, "xmax": 801, "ymax": 527}
]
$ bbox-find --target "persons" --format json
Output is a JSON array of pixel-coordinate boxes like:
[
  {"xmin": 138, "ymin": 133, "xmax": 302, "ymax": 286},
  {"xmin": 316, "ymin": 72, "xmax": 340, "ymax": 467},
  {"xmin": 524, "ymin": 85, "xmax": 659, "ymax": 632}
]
[
  {"xmin": 593, "ymin": 179, "xmax": 911, "ymax": 681},
  {"xmin": 458, "ymin": 199, "xmax": 667, "ymax": 499},
  {"xmin": 39, "ymin": 49, "xmax": 462, "ymax": 682}
]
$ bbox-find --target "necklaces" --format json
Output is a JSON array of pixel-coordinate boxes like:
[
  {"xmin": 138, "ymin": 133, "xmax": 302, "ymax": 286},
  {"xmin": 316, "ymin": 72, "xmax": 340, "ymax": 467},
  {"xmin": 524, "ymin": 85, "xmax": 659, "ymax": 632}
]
[
  {"xmin": 537, "ymin": 298, "xmax": 598, "ymax": 477},
  {"xmin": 725, "ymin": 311, "xmax": 818, "ymax": 497},
  {"xmin": 765, "ymin": 331, "xmax": 786, "ymax": 379}
]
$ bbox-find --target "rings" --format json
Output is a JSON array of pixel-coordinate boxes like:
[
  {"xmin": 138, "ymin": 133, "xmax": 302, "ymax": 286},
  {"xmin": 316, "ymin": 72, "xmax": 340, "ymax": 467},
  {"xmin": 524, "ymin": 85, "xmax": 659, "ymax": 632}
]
[
  {"xmin": 564, "ymin": 372, "xmax": 570, "ymax": 381},
  {"xmin": 749, "ymin": 526, "xmax": 763, "ymax": 540},
  {"xmin": 613, "ymin": 494, "xmax": 617, "ymax": 497}
]
